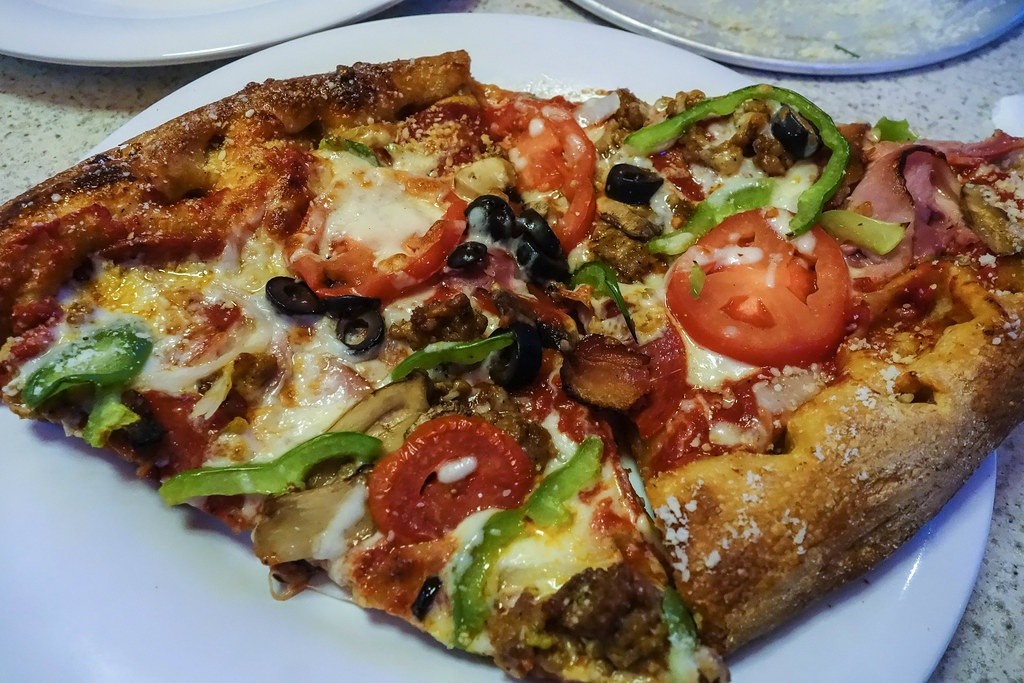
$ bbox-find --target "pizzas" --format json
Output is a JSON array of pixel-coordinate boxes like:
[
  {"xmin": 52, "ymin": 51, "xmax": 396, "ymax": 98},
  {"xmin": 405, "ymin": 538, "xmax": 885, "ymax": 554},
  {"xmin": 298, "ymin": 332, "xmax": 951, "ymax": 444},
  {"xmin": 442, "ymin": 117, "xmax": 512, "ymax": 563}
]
[{"xmin": 1, "ymin": 51, "xmax": 1023, "ymax": 683}]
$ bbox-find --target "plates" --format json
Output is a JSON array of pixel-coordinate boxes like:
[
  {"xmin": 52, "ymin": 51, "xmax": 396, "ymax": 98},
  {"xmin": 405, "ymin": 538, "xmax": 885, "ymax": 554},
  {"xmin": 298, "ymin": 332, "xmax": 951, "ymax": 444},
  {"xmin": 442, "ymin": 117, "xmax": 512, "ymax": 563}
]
[
  {"xmin": 0, "ymin": 14, "xmax": 997, "ymax": 683},
  {"xmin": 0, "ymin": 0, "xmax": 403, "ymax": 68},
  {"xmin": 564, "ymin": 0, "xmax": 1023, "ymax": 75}
]
